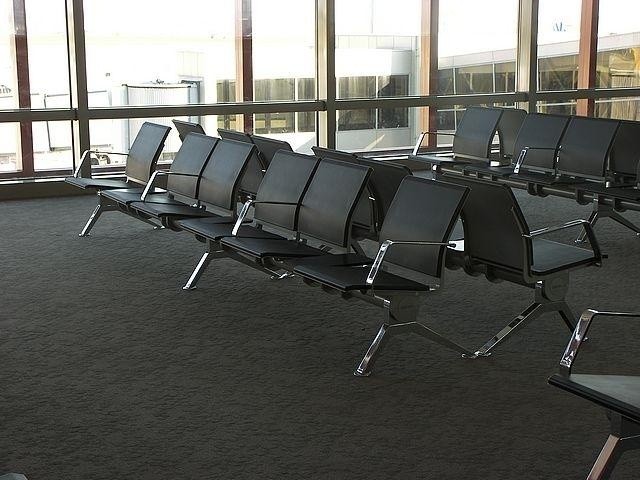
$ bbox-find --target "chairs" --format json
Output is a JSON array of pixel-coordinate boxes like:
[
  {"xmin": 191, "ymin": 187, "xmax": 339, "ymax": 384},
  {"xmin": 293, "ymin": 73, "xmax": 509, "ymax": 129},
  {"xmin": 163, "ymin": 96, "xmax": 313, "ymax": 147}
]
[{"xmin": 539, "ymin": 308, "xmax": 640, "ymax": 479}]
